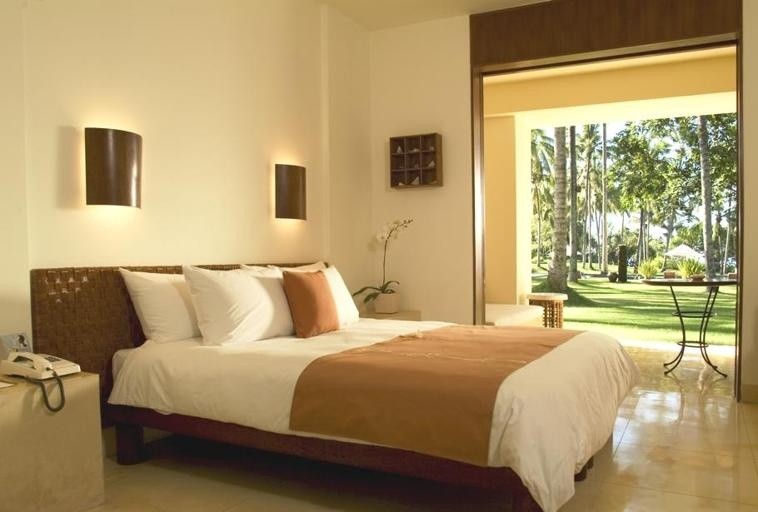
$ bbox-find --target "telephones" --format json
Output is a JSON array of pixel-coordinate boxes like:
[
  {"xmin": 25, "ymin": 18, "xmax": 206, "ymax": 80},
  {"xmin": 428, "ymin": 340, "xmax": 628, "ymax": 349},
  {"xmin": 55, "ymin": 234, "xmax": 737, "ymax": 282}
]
[{"xmin": 1, "ymin": 351, "xmax": 80, "ymax": 381}]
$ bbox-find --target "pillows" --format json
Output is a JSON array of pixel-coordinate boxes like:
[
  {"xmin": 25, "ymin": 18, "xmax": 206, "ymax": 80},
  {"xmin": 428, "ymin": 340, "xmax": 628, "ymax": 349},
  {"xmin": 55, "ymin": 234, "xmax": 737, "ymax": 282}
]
[
  {"xmin": 181, "ymin": 262, "xmax": 295, "ymax": 343},
  {"xmin": 283, "ymin": 269, "xmax": 339, "ymax": 338},
  {"xmin": 119, "ymin": 267, "xmax": 202, "ymax": 342},
  {"xmin": 324, "ymin": 265, "xmax": 360, "ymax": 324}
]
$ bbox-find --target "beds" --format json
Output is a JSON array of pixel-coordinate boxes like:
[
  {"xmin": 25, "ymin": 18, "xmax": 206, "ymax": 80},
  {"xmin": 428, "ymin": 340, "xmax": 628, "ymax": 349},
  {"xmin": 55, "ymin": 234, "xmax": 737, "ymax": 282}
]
[{"xmin": 30, "ymin": 265, "xmax": 644, "ymax": 512}]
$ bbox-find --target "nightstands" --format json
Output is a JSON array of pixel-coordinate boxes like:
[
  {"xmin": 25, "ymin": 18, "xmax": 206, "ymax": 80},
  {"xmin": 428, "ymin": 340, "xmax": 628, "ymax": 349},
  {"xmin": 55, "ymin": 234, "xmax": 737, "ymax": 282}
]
[
  {"xmin": 0, "ymin": 372, "xmax": 104, "ymax": 512},
  {"xmin": 360, "ymin": 310, "xmax": 421, "ymax": 320}
]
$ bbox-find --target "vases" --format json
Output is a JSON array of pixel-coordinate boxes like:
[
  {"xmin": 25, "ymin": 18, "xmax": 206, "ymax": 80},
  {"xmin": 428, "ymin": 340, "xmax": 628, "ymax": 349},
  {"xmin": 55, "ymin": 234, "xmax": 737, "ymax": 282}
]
[{"xmin": 375, "ymin": 292, "xmax": 402, "ymax": 314}]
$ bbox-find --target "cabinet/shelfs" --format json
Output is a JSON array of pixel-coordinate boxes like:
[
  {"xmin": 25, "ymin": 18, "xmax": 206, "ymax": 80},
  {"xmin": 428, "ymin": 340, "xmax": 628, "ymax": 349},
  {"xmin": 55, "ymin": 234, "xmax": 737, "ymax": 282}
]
[{"xmin": 389, "ymin": 133, "xmax": 443, "ymax": 188}]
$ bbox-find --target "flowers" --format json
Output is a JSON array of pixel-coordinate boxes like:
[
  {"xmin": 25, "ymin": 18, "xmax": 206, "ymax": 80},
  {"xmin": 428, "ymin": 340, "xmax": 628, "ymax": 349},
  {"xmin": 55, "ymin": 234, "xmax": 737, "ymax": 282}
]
[{"xmin": 352, "ymin": 217, "xmax": 413, "ymax": 304}]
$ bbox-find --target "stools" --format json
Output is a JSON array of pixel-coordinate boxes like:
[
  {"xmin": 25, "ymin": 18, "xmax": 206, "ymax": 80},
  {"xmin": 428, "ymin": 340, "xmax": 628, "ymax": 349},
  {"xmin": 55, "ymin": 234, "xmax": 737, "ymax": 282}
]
[{"xmin": 528, "ymin": 293, "xmax": 568, "ymax": 328}]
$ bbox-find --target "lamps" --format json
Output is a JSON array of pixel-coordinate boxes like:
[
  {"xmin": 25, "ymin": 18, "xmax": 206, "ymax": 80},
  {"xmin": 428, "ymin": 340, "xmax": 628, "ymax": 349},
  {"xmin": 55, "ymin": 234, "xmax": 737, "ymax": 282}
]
[
  {"xmin": 275, "ymin": 164, "xmax": 306, "ymax": 221},
  {"xmin": 84, "ymin": 126, "xmax": 143, "ymax": 209}
]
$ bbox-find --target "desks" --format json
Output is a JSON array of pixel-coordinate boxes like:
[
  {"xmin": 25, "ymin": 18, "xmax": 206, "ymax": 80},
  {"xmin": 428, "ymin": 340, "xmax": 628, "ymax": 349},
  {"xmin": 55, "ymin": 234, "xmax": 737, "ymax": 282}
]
[{"xmin": 642, "ymin": 275, "xmax": 737, "ymax": 378}]
